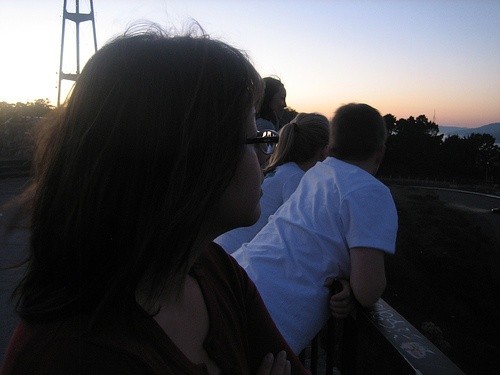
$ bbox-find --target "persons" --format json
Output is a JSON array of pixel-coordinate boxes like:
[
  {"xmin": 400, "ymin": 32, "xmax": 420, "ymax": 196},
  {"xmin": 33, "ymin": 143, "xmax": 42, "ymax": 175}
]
[
  {"xmin": 213, "ymin": 113, "xmax": 332, "ymax": 254},
  {"xmin": 256, "ymin": 78, "xmax": 287, "ymax": 160},
  {"xmin": 229, "ymin": 103, "xmax": 398, "ymax": 355},
  {"xmin": 0, "ymin": 34, "xmax": 309, "ymax": 375}
]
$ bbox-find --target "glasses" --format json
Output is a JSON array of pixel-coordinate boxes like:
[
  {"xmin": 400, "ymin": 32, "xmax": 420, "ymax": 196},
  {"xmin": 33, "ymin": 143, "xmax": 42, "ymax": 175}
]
[{"xmin": 241, "ymin": 129, "xmax": 280, "ymax": 155}]
